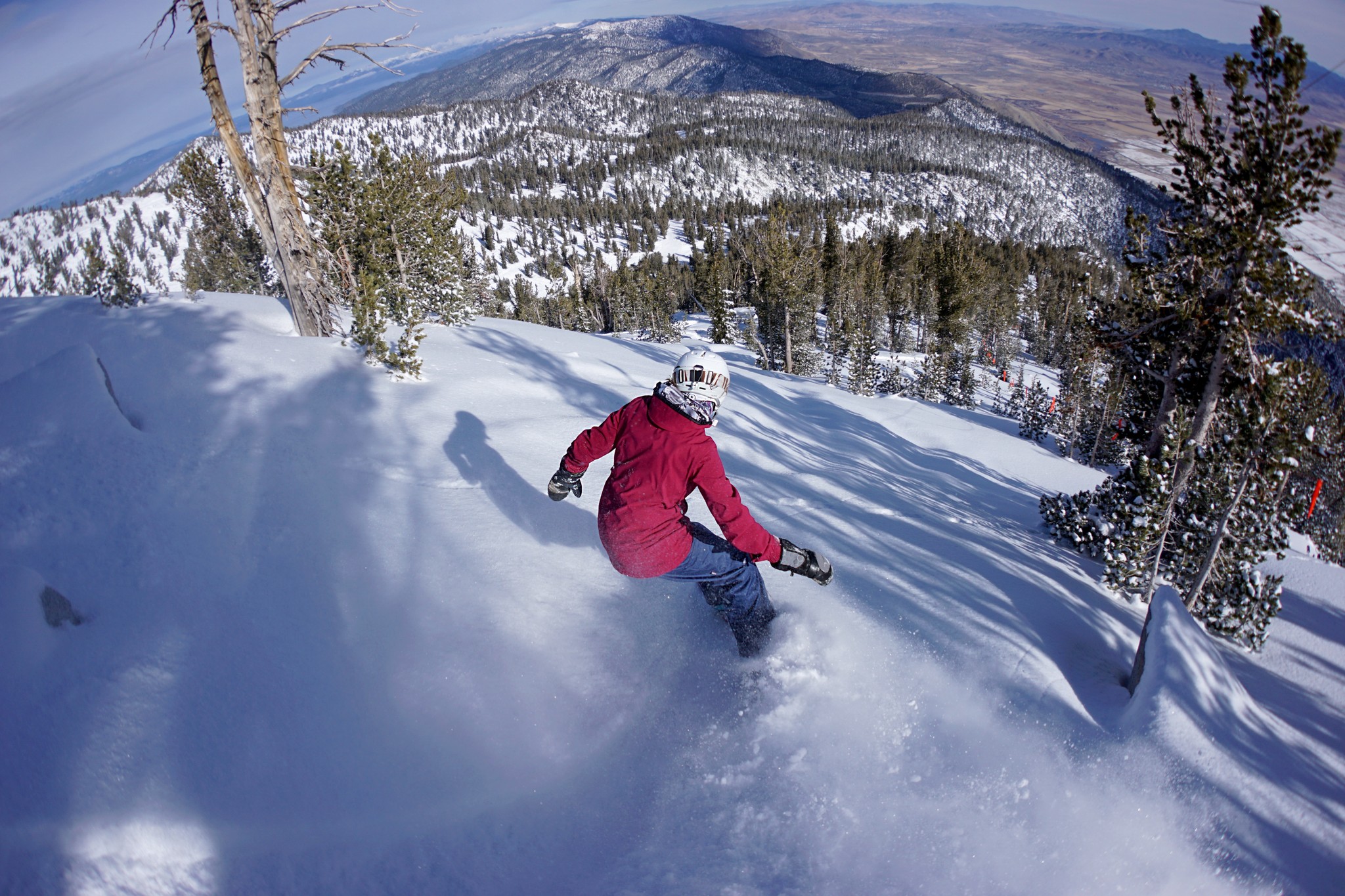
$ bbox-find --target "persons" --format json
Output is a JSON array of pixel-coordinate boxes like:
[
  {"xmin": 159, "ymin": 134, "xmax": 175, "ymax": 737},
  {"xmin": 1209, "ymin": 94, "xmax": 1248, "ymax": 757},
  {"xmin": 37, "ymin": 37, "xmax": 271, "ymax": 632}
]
[{"xmin": 547, "ymin": 346, "xmax": 838, "ymax": 662}]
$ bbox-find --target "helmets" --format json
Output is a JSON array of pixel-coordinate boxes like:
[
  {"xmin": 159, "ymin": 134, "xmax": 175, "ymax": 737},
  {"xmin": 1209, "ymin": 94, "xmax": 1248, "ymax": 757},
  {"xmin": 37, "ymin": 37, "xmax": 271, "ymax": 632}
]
[{"xmin": 672, "ymin": 349, "xmax": 730, "ymax": 408}]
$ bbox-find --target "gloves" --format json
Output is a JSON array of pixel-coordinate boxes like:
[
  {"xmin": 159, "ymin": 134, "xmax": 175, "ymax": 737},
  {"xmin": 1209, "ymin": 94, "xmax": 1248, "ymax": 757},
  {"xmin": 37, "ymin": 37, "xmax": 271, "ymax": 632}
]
[
  {"xmin": 769, "ymin": 536, "xmax": 834, "ymax": 588},
  {"xmin": 547, "ymin": 466, "xmax": 582, "ymax": 502}
]
